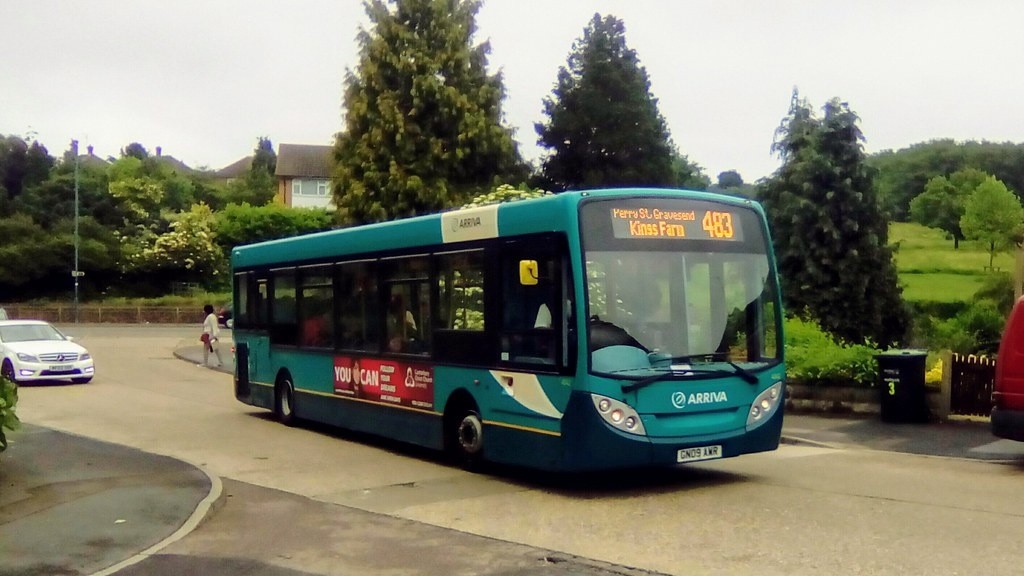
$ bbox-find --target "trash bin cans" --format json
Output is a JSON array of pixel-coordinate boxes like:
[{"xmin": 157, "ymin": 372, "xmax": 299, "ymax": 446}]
[{"xmin": 870, "ymin": 347, "xmax": 929, "ymax": 426}]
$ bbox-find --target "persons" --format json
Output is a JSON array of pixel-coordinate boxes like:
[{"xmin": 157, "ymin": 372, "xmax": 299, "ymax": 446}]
[
  {"xmin": 389, "ymin": 335, "xmax": 403, "ymax": 353},
  {"xmin": 197, "ymin": 304, "xmax": 224, "ymax": 368},
  {"xmin": 303, "ymin": 310, "xmax": 332, "ymax": 350},
  {"xmin": 387, "ymin": 293, "xmax": 422, "ymax": 355}
]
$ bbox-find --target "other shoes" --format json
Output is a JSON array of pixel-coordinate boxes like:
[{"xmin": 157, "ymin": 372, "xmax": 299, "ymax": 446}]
[
  {"xmin": 215, "ymin": 363, "xmax": 223, "ymax": 367},
  {"xmin": 197, "ymin": 363, "xmax": 207, "ymax": 368}
]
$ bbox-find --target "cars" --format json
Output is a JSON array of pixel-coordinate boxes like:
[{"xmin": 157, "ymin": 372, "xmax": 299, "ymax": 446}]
[
  {"xmin": 1, "ymin": 320, "xmax": 95, "ymax": 385},
  {"xmin": 218, "ymin": 302, "xmax": 234, "ymax": 329},
  {"xmin": 988, "ymin": 296, "xmax": 1024, "ymax": 443}
]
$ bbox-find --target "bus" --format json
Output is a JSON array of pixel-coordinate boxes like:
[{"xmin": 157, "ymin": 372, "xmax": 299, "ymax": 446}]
[{"xmin": 230, "ymin": 187, "xmax": 787, "ymax": 475}]
